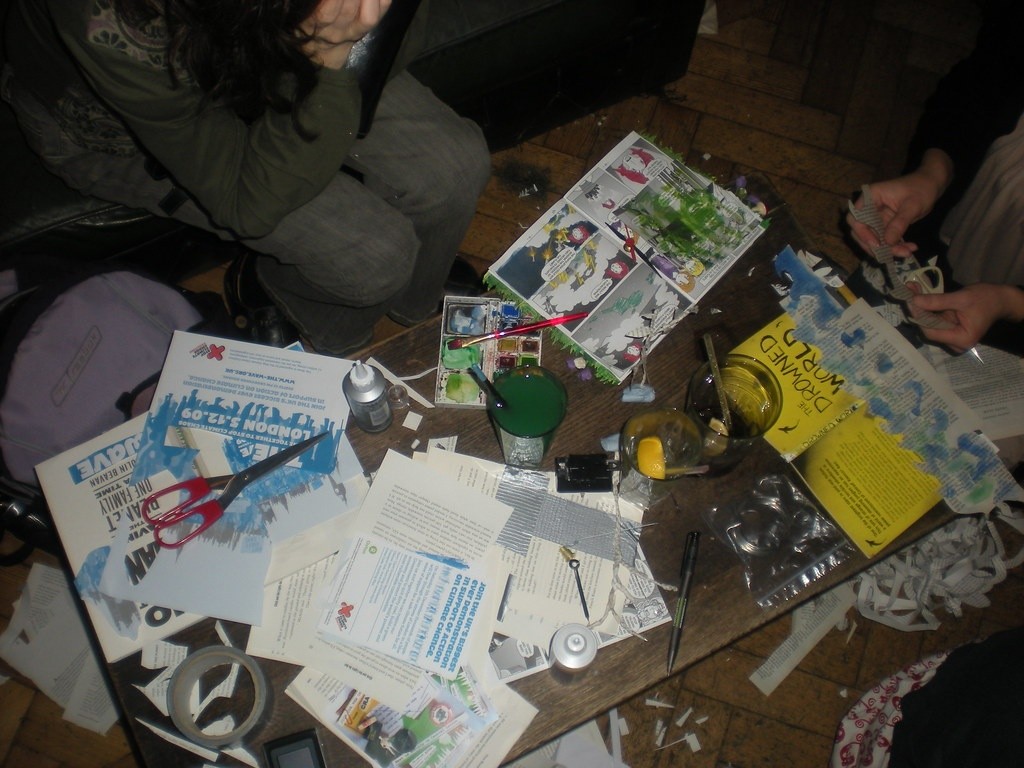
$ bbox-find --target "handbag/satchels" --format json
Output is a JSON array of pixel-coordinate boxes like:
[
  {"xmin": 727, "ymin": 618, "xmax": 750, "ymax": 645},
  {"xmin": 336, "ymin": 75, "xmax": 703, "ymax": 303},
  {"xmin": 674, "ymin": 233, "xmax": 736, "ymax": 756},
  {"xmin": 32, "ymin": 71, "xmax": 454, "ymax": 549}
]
[{"xmin": 0, "ymin": 271, "xmax": 205, "ymax": 488}]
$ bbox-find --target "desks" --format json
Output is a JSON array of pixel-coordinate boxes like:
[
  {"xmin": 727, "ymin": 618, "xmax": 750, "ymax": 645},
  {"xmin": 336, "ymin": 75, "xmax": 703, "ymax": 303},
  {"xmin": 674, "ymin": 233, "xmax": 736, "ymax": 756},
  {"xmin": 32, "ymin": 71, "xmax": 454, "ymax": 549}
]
[{"xmin": 47, "ymin": 176, "xmax": 1023, "ymax": 768}]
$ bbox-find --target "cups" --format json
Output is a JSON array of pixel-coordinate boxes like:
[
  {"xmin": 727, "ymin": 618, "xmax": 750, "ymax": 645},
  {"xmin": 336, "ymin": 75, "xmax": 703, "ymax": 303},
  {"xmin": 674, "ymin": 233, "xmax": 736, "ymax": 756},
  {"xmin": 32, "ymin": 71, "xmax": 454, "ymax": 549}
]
[
  {"xmin": 490, "ymin": 365, "xmax": 568, "ymax": 467},
  {"xmin": 685, "ymin": 354, "xmax": 783, "ymax": 477},
  {"xmin": 620, "ymin": 406, "xmax": 701, "ymax": 510}
]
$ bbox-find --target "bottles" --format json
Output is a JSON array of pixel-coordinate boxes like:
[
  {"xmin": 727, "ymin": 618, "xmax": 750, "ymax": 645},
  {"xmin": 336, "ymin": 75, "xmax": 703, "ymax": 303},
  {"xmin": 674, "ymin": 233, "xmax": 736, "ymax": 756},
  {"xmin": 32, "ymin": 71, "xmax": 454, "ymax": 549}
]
[
  {"xmin": 342, "ymin": 360, "xmax": 393, "ymax": 433},
  {"xmin": 548, "ymin": 623, "xmax": 597, "ymax": 685}
]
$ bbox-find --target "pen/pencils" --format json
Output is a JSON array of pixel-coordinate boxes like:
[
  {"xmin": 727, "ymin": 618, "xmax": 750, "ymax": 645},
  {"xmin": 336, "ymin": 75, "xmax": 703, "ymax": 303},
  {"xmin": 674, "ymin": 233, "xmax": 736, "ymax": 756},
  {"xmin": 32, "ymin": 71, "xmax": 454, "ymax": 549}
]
[{"xmin": 665, "ymin": 530, "xmax": 702, "ymax": 677}]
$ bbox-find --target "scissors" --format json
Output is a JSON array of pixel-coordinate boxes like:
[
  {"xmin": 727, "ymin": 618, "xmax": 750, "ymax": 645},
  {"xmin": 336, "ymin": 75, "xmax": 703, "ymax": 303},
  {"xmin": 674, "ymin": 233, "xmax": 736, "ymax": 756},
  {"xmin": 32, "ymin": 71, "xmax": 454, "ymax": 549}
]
[
  {"xmin": 906, "ymin": 266, "xmax": 985, "ymax": 365},
  {"xmin": 141, "ymin": 430, "xmax": 332, "ymax": 550}
]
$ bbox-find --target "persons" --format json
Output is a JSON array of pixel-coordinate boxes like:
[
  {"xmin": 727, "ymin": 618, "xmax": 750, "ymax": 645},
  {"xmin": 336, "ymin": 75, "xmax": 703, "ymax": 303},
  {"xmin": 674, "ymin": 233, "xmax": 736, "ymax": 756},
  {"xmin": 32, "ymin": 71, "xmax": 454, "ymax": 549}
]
[
  {"xmin": 848, "ymin": 0, "xmax": 1024, "ymax": 355},
  {"xmin": 0, "ymin": 0, "xmax": 494, "ymax": 359}
]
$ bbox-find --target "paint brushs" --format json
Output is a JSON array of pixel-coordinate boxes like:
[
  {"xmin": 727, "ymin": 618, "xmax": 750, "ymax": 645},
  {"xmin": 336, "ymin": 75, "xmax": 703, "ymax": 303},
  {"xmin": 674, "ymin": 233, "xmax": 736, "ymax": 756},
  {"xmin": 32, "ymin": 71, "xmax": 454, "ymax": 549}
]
[
  {"xmin": 470, "ymin": 362, "xmax": 509, "ymax": 411},
  {"xmin": 446, "ymin": 309, "xmax": 589, "ymax": 351}
]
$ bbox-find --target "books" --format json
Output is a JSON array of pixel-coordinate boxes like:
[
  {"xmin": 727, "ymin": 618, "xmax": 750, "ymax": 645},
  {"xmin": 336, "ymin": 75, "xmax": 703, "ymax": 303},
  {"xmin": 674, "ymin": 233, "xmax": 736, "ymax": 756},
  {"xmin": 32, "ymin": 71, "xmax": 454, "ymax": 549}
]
[
  {"xmin": 721, "ymin": 276, "xmax": 1000, "ymax": 562},
  {"xmin": 483, "ymin": 131, "xmax": 771, "ymax": 387}
]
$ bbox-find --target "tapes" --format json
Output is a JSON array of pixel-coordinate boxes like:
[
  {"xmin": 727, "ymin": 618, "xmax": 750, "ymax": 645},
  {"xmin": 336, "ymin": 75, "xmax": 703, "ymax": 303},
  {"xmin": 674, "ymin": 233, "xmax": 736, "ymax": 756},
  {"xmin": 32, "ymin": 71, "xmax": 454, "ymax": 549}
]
[{"xmin": 163, "ymin": 644, "xmax": 278, "ymax": 753}]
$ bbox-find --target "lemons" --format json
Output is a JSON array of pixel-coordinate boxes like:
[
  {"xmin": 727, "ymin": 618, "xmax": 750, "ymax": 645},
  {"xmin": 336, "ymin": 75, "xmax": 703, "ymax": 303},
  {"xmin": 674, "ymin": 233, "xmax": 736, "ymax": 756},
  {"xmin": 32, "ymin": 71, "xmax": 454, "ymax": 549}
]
[
  {"xmin": 637, "ymin": 436, "xmax": 666, "ymax": 479},
  {"xmin": 703, "ymin": 417, "xmax": 729, "ymax": 457}
]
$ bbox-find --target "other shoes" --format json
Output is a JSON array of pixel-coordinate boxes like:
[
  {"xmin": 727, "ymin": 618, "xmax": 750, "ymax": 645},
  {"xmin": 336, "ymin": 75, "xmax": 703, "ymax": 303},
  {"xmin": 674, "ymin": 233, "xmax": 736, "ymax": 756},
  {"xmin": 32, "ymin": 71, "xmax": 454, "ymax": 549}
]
[{"xmin": 224, "ymin": 248, "xmax": 303, "ymax": 351}]
